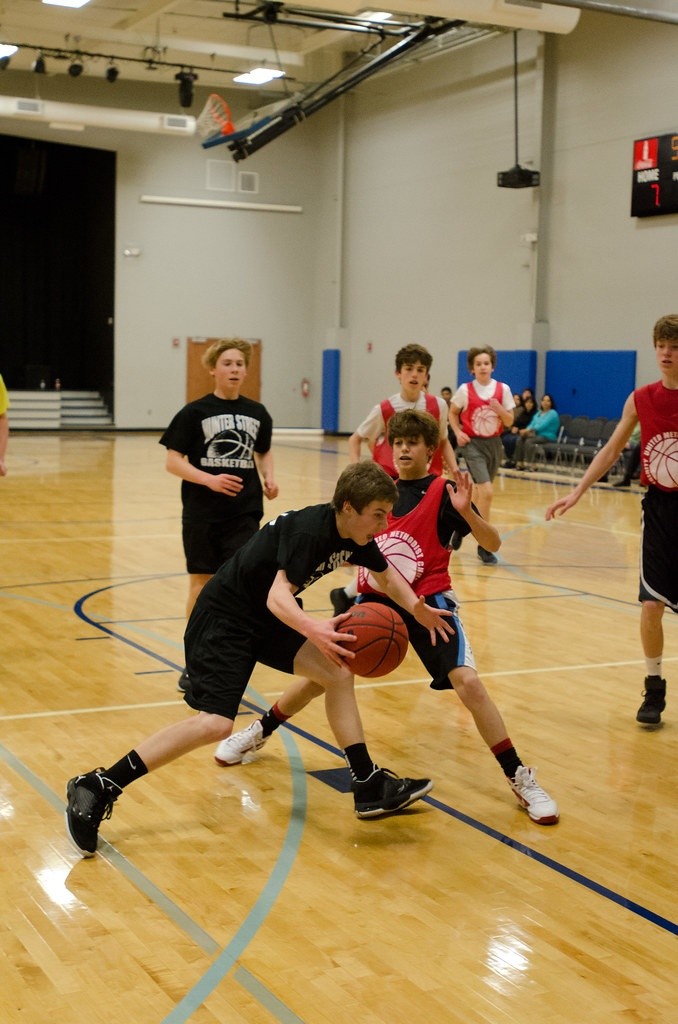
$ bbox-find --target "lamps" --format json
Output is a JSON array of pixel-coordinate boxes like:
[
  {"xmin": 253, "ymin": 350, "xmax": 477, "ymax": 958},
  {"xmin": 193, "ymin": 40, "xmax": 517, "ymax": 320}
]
[
  {"xmin": 106, "ymin": 58, "xmax": 119, "ymax": 83},
  {"xmin": 32, "ymin": 49, "xmax": 49, "ymax": 73},
  {"xmin": 175, "ymin": 67, "xmax": 198, "ymax": 108},
  {"xmin": 68, "ymin": 54, "xmax": 84, "ymax": 77}
]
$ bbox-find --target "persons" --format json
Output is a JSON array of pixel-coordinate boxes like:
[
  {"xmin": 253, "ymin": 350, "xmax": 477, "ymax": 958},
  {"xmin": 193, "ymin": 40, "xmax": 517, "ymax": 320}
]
[
  {"xmin": 159, "ymin": 336, "xmax": 278, "ymax": 692},
  {"xmin": 545, "ymin": 313, "xmax": 678, "ymax": 726},
  {"xmin": 65, "ymin": 460, "xmax": 456, "ymax": 856},
  {"xmin": 350, "ymin": 340, "xmax": 640, "ymax": 565},
  {"xmin": 214, "ymin": 408, "xmax": 562, "ymax": 825},
  {"xmin": 0, "ymin": 376, "xmax": 9, "ymax": 477}
]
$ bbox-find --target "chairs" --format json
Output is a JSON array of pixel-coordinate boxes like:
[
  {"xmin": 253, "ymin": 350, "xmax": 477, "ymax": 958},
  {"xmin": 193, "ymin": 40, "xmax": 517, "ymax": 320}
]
[{"xmin": 531, "ymin": 416, "xmax": 627, "ymax": 480}]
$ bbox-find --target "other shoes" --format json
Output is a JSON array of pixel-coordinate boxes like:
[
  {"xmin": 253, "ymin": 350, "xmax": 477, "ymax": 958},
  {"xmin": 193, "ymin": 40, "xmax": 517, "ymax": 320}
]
[
  {"xmin": 502, "ymin": 461, "xmax": 516, "ymax": 468},
  {"xmin": 516, "ymin": 466, "xmax": 538, "ymax": 472},
  {"xmin": 613, "ymin": 481, "xmax": 631, "ymax": 487}
]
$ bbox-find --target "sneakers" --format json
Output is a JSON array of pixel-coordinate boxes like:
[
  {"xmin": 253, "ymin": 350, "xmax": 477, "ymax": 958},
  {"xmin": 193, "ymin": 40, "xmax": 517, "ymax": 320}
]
[
  {"xmin": 66, "ymin": 767, "xmax": 123, "ymax": 858},
  {"xmin": 476, "ymin": 544, "xmax": 498, "ymax": 565},
  {"xmin": 451, "ymin": 532, "xmax": 462, "ymax": 550},
  {"xmin": 214, "ymin": 719, "xmax": 271, "ymax": 766},
  {"xmin": 353, "ymin": 770, "xmax": 434, "ymax": 819},
  {"xmin": 504, "ymin": 766, "xmax": 560, "ymax": 825},
  {"xmin": 636, "ymin": 678, "xmax": 666, "ymax": 725}
]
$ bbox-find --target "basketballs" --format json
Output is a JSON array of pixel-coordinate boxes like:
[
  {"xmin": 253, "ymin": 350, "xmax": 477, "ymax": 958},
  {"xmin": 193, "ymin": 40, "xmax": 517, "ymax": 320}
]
[{"xmin": 336, "ymin": 602, "xmax": 410, "ymax": 679}]
